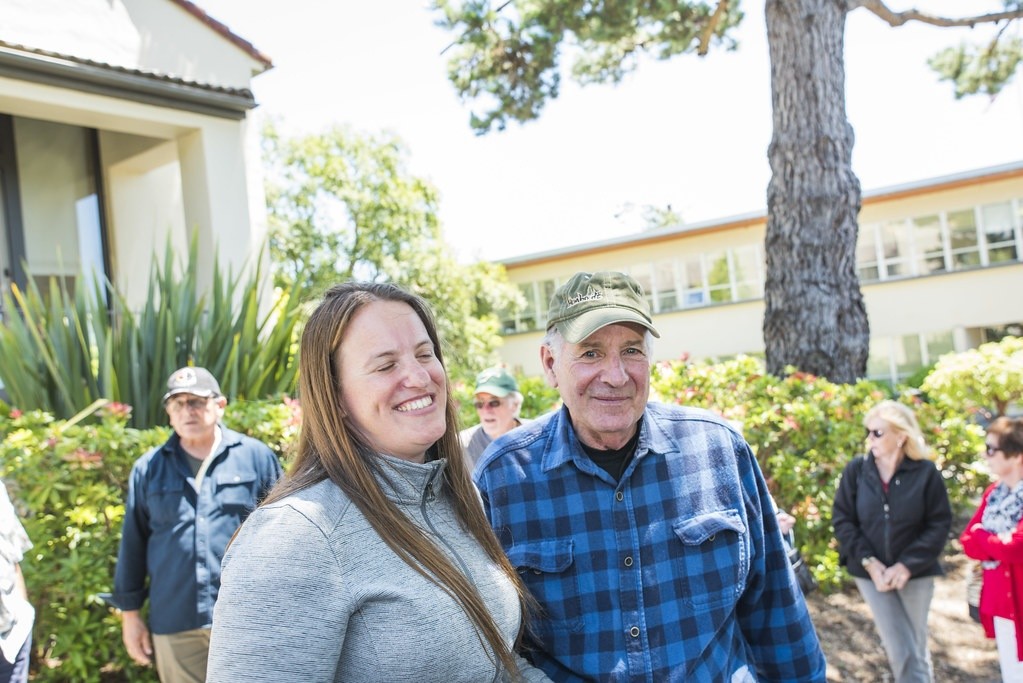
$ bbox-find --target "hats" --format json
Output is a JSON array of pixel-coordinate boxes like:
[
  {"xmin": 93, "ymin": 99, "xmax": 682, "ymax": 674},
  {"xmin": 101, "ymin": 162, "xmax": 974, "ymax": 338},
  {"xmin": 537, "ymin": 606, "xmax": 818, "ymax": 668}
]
[
  {"xmin": 476, "ymin": 366, "xmax": 517, "ymax": 398},
  {"xmin": 545, "ymin": 271, "xmax": 660, "ymax": 343},
  {"xmin": 162, "ymin": 366, "xmax": 221, "ymax": 401}
]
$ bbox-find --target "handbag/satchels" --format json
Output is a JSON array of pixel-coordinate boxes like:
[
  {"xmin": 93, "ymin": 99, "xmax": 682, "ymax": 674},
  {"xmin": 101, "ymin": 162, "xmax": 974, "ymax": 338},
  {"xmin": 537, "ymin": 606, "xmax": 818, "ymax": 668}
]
[{"xmin": 968, "ymin": 563, "xmax": 982, "ymax": 622}]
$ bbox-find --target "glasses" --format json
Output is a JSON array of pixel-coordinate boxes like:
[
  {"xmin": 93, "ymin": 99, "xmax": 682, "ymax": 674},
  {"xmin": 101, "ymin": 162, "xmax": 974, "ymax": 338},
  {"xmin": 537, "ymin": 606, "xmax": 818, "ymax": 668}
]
[
  {"xmin": 474, "ymin": 399, "xmax": 507, "ymax": 409},
  {"xmin": 866, "ymin": 428, "xmax": 893, "ymax": 437},
  {"xmin": 985, "ymin": 444, "xmax": 1003, "ymax": 456}
]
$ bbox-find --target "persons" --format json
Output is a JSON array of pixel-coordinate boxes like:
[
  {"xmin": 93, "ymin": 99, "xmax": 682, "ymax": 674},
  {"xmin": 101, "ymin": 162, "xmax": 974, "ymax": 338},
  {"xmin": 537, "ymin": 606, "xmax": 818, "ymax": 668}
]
[
  {"xmin": 99, "ymin": 365, "xmax": 285, "ymax": 683},
  {"xmin": 452, "ymin": 363, "xmax": 536, "ymax": 480},
  {"xmin": 204, "ymin": 278, "xmax": 554, "ymax": 683},
  {"xmin": 830, "ymin": 399, "xmax": 952, "ymax": 682},
  {"xmin": 960, "ymin": 415, "xmax": 1023, "ymax": 683},
  {"xmin": 0, "ymin": 478, "xmax": 35, "ymax": 683},
  {"xmin": 469, "ymin": 270, "xmax": 833, "ymax": 682}
]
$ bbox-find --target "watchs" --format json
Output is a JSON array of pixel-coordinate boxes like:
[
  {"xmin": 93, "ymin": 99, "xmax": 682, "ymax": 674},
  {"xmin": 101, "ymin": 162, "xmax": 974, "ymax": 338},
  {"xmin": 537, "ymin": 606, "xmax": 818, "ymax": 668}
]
[{"xmin": 860, "ymin": 557, "xmax": 875, "ymax": 567}]
